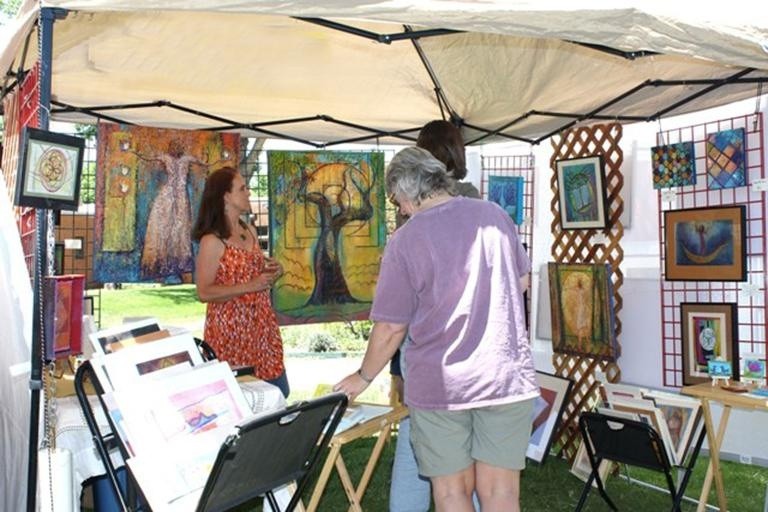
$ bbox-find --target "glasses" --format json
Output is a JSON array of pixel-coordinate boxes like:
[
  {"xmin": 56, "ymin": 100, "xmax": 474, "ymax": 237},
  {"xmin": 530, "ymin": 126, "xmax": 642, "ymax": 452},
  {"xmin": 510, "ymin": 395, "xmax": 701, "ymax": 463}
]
[{"xmin": 389, "ymin": 192, "xmax": 402, "ymax": 207}]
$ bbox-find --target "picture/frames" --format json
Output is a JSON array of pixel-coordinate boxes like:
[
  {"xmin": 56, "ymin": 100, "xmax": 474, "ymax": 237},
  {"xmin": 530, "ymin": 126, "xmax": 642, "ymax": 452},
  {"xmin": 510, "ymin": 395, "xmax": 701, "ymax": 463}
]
[
  {"xmin": 525, "ymin": 370, "xmax": 575, "ymax": 466},
  {"xmin": 556, "ymin": 155, "xmax": 606, "ymax": 230},
  {"xmin": 680, "ymin": 302, "xmax": 739, "ymax": 386},
  {"xmin": 664, "ymin": 206, "xmax": 747, "ymax": 283},
  {"xmin": 14, "ymin": 126, "xmax": 85, "ymax": 211}
]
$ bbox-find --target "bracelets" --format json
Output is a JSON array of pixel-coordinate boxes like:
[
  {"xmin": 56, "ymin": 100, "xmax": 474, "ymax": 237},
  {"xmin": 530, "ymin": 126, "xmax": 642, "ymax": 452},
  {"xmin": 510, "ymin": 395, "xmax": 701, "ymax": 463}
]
[{"xmin": 356, "ymin": 367, "xmax": 373, "ymax": 384}]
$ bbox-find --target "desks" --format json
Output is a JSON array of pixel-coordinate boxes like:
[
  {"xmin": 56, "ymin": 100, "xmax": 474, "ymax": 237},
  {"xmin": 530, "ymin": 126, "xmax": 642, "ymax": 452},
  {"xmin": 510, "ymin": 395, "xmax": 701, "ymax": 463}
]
[{"xmin": 290, "ymin": 401, "xmax": 409, "ymax": 512}]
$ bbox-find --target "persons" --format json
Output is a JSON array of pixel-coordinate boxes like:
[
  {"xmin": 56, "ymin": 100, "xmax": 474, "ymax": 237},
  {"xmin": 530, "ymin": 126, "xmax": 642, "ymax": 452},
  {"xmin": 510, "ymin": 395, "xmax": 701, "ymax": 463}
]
[
  {"xmin": 388, "ymin": 120, "xmax": 481, "ymax": 512},
  {"xmin": 693, "ymin": 222, "xmax": 712, "ymax": 256},
  {"xmin": 561, "ymin": 275, "xmax": 601, "ymax": 352},
  {"xmin": 332, "ymin": 146, "xmax": 542, "ymax": 512},
  {"xmin": 190, "ymin": 166, "xmax": 290, "ymax": 399},
  {"xmin": 126, "ymin": 138, "xmax": 236, "ymax": 284}
]
